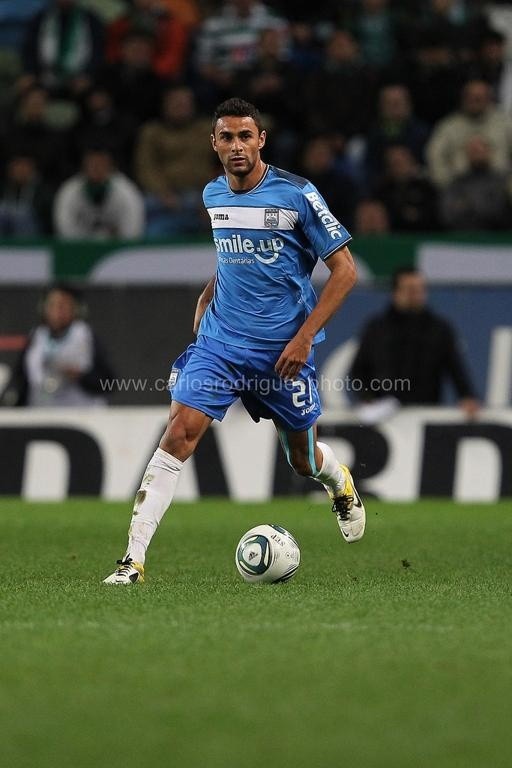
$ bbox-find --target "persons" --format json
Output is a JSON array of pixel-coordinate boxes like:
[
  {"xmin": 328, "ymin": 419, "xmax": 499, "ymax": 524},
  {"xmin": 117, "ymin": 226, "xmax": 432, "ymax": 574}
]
[
  {"xmin": 0, "ymin": 285, "xmax": 114, "ymax": 407},
  {"xmin": 98, "ymin": 97, "xmax": 367, "ymax": 584},
  {"xmin": 0, "ymin": 0, "xmax": 512, "ymax": 241},
  {"xmin": 346, "ymin": 267, "xmax": 480, "ymax": 423}
]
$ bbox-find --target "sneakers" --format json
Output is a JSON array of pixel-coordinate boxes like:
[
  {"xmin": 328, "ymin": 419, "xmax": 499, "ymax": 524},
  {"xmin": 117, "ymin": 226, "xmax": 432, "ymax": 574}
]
[
  {"xmin": 103, "ymin": 553, "xmax": 145, "ymax": 585},
  {"xmin": 323, "ymin": 465, "xmax": 367, "ymax": 544}
]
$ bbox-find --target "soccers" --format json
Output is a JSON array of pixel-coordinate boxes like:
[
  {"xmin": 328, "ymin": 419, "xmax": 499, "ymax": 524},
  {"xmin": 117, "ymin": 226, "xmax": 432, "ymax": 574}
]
[{"xmin": 234, "ymin": 523, "xmax": 301, "ymax": 586}]
